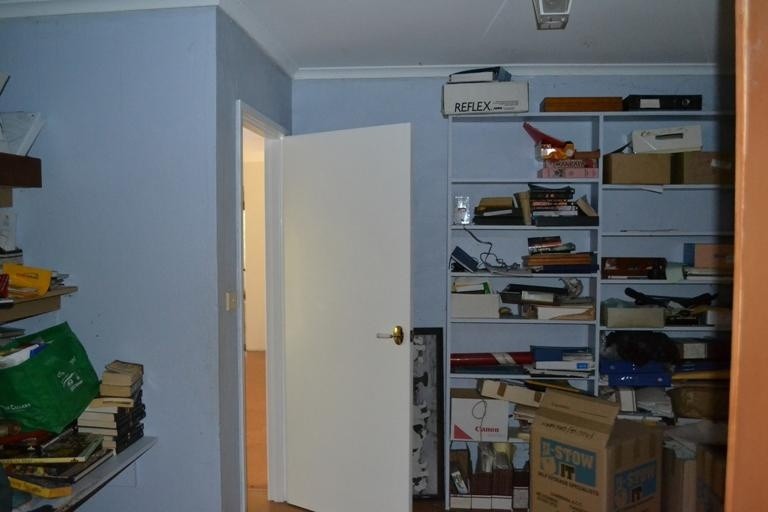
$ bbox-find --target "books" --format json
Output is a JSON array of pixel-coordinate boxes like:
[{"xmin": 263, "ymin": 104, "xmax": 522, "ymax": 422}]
[
  {"xmin": 0, "ymin": 360, "xmax": 149, "ymax": 499},
  {"xmin": 477, "ymin": 184, "xmax": 581, "ymax": 220}
]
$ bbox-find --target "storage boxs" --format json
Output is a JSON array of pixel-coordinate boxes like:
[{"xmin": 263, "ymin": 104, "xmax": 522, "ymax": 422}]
[{"xmin": 441, "ymin": 81, "xmax": 703, "ymax": 115}]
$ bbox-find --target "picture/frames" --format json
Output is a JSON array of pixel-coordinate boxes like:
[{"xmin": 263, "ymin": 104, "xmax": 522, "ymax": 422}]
[{"xmin": 413, "ymin": 327, "xmax": 443, "ymax": 500}]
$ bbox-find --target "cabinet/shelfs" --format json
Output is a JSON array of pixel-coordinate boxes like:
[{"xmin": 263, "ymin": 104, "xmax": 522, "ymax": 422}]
[
  {"xmin": 443, "ymin": 109, "xmax": 730, "ymax": 511},
  {"xmin": 0, "ymin": 144, "xmax": 158, "ymax": 512}
]
